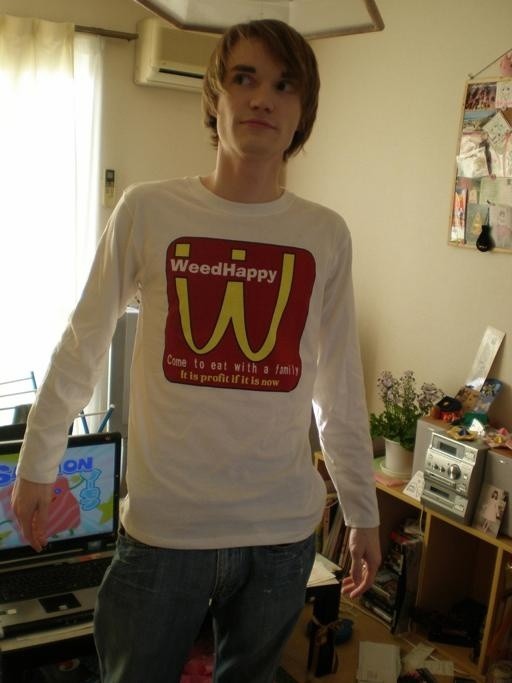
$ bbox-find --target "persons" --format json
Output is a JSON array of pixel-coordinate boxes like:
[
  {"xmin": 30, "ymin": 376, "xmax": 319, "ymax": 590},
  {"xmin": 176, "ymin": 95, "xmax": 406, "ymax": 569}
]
[{"xmin": 9, "ymin": 19, "xmax": 382, "ymax": 683}]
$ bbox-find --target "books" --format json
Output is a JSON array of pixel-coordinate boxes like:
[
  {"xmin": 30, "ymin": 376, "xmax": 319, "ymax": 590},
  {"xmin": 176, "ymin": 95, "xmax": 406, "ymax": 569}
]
[
  {"xmin": 314, "ymin": 479, "xmax": 351, "ymax": 572},
  {"xmin": 363, "ymin": 517, "xmax": 426, "ymax": 614}
]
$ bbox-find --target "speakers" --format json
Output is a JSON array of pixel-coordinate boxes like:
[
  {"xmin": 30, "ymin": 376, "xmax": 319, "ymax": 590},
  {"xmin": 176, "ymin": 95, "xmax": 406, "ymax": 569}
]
[
  {"xmin": 411, "ymin": 414, "xmax": 458, "ymax": 482},
  {"xmin": 483, "ymin": 447, "xmax": 512, "ymax": 537}
]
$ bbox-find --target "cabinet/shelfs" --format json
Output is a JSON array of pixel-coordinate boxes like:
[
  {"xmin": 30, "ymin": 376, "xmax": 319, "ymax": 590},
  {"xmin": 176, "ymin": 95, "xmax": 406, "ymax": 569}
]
[{"xmin": 311, "ymin": 452, "xmax": 512, "ymax": 683}]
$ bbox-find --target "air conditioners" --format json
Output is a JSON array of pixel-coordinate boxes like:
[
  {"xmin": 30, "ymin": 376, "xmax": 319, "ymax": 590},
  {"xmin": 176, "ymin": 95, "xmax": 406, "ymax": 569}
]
[{"xmin": 133, "ymin": 16, "xmax": 224, "ymax": 91}]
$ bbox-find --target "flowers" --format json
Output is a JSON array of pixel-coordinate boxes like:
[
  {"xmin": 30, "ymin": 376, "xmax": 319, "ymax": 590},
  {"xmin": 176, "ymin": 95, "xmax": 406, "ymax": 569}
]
[{"xmin": 368, "ymin": 368, "xmax": 446, "ymax": 451}]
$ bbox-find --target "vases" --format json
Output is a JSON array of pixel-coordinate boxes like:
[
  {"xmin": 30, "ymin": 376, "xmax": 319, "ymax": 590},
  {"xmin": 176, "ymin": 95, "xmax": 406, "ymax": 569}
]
[{"xmin": 381, "ymin": 437, "xmax": 413, "ymax": 476}]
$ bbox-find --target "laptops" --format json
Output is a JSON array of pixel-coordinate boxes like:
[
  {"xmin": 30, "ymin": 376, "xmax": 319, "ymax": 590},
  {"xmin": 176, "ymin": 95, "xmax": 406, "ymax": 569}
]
[{"xmin": 0, "ymin": 431, "xmax": 122, "ymax": 638}]
